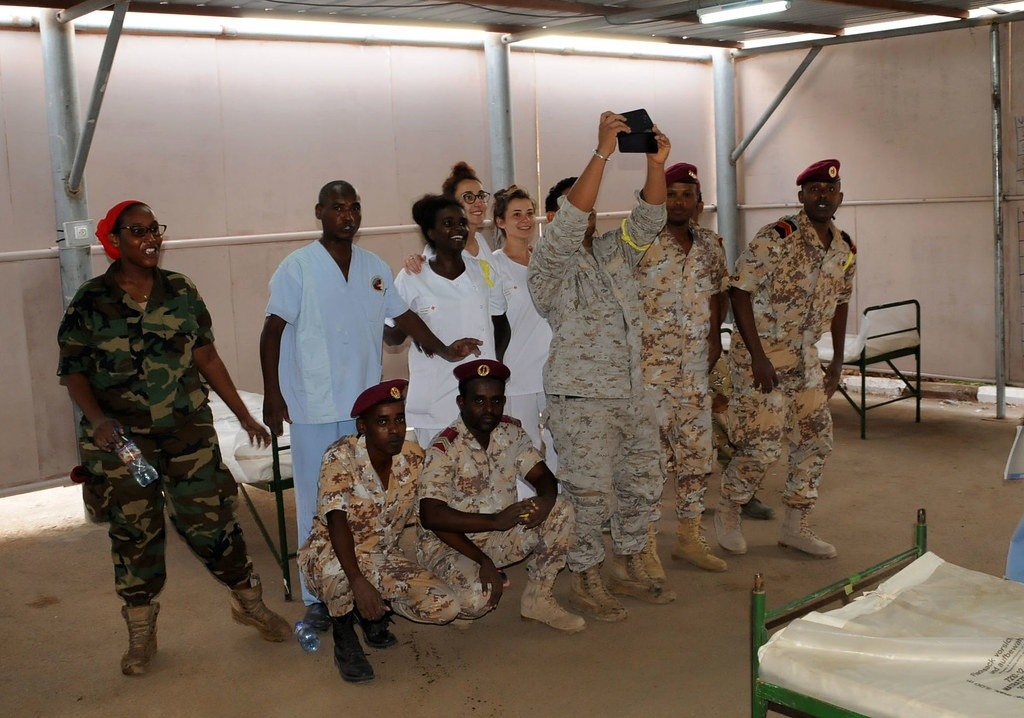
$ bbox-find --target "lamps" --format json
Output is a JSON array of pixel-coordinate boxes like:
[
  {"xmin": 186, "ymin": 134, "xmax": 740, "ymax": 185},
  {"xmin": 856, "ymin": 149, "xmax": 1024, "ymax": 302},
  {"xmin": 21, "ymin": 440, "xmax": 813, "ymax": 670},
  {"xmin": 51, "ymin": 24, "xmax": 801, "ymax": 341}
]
[{"xmin": 696, "ymin": 0, "xmax": 792, "ymax": 24}]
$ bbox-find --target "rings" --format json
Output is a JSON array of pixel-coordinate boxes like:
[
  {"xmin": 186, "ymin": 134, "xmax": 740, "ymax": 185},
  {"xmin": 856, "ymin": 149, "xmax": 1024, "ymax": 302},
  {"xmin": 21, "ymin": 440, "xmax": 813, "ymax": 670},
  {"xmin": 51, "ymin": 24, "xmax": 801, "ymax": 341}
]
[{"xmin": 410, "ymin": 257, "xmax": 413, "ymax": 260}]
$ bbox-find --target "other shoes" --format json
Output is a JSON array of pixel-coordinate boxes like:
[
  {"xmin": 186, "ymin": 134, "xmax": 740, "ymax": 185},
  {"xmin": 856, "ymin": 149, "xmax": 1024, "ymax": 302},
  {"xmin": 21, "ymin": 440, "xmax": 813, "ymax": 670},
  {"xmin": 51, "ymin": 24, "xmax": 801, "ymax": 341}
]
[{"xmin": 740, "ymin": 492, "xmax": 775, "ymax": 520}]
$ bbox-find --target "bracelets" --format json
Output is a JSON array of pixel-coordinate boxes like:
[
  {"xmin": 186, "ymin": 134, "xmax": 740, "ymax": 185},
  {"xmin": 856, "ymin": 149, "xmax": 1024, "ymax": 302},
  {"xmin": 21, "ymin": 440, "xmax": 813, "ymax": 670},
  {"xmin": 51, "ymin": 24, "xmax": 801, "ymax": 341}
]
[{"xmin": 593, "ymin": 148, "xmax": 610, "ymax": 161}]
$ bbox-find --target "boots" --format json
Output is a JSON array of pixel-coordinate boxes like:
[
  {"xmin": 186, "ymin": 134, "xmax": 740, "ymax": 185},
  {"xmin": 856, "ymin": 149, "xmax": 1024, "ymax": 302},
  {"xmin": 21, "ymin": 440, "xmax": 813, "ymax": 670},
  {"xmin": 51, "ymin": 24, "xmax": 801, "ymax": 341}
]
[
  {"xmin": 713, "ymin": 498, "xmax": 747, "ymax": 554},
  {"xmin": 778, "ymin": 506, "xmax": 836, "ymax": 559},
  {"xmin": 568, "ymin": 563, "xmax": 629, "ymax": 621},
  {"xmin": 671, "ymin": 515, "xmax": 727, "ymax": 572},
  {"xmin": 637, "ymin": 521, "xmax": 667, "ymax": 583},
  {"xmin": 227, "ymin": 572, "xmax": 292, "ymax": 641},
  {"xmin": 333, "ymin": 612, "xmax": 374, "ymax": 683},
  {"xmin": 607, "ymin": 552, "xmax": 677, "ymax": 604},
  {"xmin": 353, "ymin": 602, "xmax": 398, "ymax": 648},
  {"xmin": 521, "ymin": 580, "xmax": 586, "ymax": 633},
  {"xmin": 120, "ymin": 600, "xmax": 160, "ymax": 675}
]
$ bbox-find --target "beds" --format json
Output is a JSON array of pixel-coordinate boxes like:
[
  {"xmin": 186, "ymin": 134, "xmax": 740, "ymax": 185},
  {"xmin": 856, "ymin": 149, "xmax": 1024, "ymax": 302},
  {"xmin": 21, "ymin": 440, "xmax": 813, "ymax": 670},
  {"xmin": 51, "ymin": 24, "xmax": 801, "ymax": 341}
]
[
  {"xmin": 722, "ymin": 299, "xmax": 922, "ymax": 439},
  {"xmin": 750, "ymin": 509, "xmax": 1024, "ymax": 718},
  {"xmin": 205, "ymin": 389, "xmax": 298, "ymax": 601}
]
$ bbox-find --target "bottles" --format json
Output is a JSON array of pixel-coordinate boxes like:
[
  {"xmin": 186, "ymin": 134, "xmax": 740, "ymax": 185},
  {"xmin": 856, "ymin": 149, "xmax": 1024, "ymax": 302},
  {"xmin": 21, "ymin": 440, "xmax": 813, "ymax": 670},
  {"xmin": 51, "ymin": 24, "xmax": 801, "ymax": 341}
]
[
  {"xmin": 294, "ymin": 620, "xmax": 322, "ymax": 653},
  {"xmin": 112, "ymin": 427, "xmax": 159, "ymax": 488}
]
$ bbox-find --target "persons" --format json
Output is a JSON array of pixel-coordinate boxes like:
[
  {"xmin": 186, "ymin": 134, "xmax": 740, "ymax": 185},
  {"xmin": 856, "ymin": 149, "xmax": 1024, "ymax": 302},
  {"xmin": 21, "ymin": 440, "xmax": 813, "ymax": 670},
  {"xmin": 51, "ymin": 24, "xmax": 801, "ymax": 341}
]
[
  {"xmin": 714, "ymin": 160, "xmax": 857, "ymax": 558},
  {"xmin": 405, "ymin": 162, "xmax": 561, "ymax": 501},
  {"xmin": 636, "ymin": 162, "xmax": 727, "ymax": 582},
  {"xmin": 298, "ymin": 379, "xmax": 460, "ymax": 682},
  {"xmin": 419, "ymin": 359, "xmax": 586, "ymax": 633},
  {"xmin": 56, "ymin": 199, "xmax": 293, "ymax": 677},
  {"xmin": 259, "ymin": 180, "xmax": 482, "ymax": 633},
  {"xmin": 383, "ymin": 194, "xmax": 512, "ymax": 451},
  {"xmin": 526, "ymin": 108, "xmax": 677, "ymax": 622}
]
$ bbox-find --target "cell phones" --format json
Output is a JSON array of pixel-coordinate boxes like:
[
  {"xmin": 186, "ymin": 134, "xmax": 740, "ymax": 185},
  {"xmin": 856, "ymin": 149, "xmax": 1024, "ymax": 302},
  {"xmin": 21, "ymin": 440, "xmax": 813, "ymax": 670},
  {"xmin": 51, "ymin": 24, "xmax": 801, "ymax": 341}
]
[{"xmin": 615, "ymin": 109, "xmax": 658, "ymax": 153}]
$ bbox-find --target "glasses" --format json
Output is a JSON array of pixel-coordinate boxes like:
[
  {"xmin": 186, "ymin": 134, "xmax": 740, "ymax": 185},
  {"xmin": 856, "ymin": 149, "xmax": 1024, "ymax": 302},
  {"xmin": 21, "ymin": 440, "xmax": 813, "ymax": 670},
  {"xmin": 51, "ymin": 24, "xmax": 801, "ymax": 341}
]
[
  {"xmin": 455, "ymin": 192, "xmax": 490, "ymax": 204},
  {"xmin": 118, "ymin": 224, "xmax": 167, "ymax": 238}
]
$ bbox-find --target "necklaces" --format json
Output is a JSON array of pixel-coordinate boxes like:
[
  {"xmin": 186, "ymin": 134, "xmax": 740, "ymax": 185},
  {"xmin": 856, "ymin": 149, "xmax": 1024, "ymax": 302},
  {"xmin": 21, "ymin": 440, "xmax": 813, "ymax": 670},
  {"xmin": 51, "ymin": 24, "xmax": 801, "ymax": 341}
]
[{"xmin": 144, "ymin": 295, "xmax": 147, "ymax": 298}]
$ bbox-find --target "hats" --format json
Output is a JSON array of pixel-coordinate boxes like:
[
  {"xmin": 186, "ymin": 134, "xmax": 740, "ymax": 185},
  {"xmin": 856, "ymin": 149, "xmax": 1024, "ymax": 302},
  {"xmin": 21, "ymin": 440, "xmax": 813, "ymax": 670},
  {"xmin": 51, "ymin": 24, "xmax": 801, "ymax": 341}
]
[
  {"xmin": 796, "ymin": 158, "xmax": 841, "ymax": 185},
  {"xmin": 350, "ymin": 379, "xmax": 409, "ymax": 418},
  {"xmin": 662, "ymin": 162, "xmax": 699, "ymax": 186},
  {"xmin": 453, "ymin": 359, "xmax": 510, "ymax": 383}
]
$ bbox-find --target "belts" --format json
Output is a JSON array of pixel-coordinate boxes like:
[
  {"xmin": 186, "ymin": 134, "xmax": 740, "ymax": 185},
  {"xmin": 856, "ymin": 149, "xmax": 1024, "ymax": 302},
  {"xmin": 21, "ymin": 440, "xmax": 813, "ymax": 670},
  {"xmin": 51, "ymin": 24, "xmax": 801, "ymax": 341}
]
[{"xmin": 549, "ymin": 391, "xmax": 586, "ymax": 399}]
